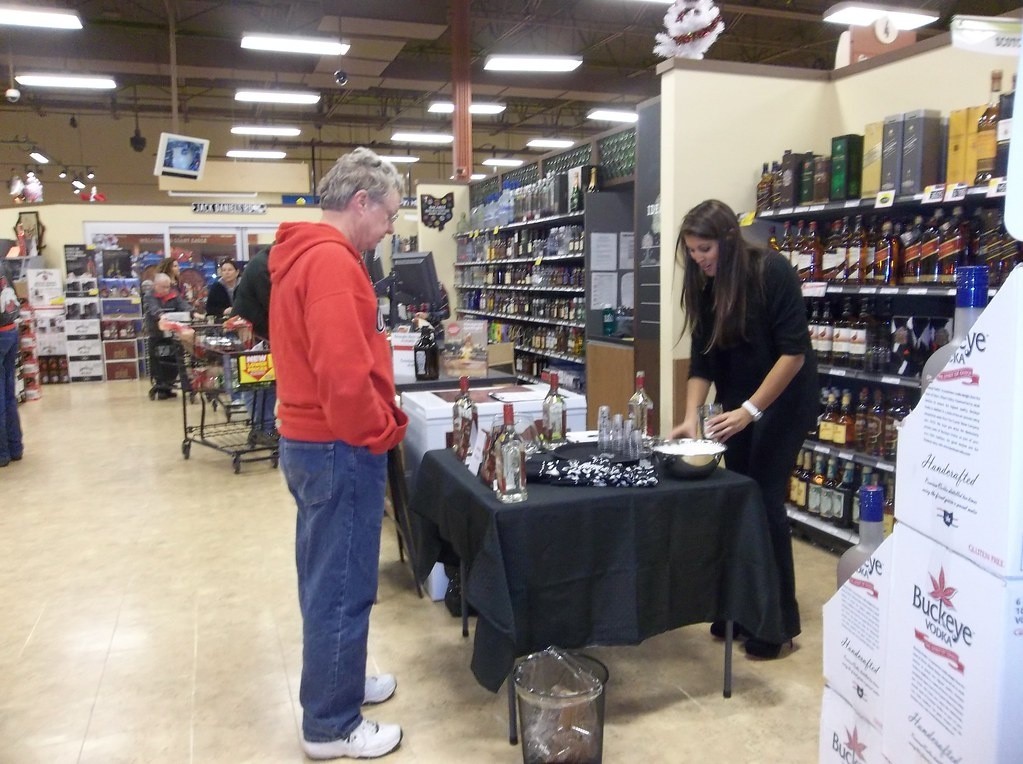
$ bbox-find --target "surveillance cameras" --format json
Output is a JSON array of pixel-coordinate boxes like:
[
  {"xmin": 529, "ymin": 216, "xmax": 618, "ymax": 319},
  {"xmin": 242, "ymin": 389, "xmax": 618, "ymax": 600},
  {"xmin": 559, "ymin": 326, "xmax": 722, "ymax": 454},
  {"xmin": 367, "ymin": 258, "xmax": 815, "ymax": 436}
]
[
  {"xmin": 334, "ymin": 71, "xmax": 347, "ymax": 86},
  {"xmin": 129, "ymin": 136, "xmax": 146, "ymax": 152},
  {"xmin": 5, "ymin": 89, "xmax": 20, "ymax": 102}
]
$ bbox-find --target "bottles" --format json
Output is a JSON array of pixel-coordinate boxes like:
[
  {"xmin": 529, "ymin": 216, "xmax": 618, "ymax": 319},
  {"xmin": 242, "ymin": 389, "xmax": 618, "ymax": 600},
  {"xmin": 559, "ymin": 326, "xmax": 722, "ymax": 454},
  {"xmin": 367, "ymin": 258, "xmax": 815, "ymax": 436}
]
[
  {"xmin": 496, "ymin": 404, "xmax": 528, "ymax": 503},
  {"xmin": 542, "ymin": 372, "xmax": 567, "ymax": 449},
  {"xmin": 453, "ymin": 375, "xmax": 477, "ymax": 452},
  {"xmin": 602, "ymin": 304, "xmax": 615, "ymax": 336},
  {"xmin": 627, "ymin": 371, "xmax": 653, "ymax": 448},
  {"xmin": 837, "ymin": 483, "xmax": 887, "ymax": 592},
  {"xmin": 414, "ymin": 326, "xmax": 439, "ymax": 380},
  {"xmin": 751, "ymin": 150, "xmax": 1022, "ymax": 541},
  {"xmin": 456, "ymin": 168, "xmax": 598, "ymax": 378},
  {"xmin": 975, "ymin": 69, "xmax": 1003, "ymax": 186}
]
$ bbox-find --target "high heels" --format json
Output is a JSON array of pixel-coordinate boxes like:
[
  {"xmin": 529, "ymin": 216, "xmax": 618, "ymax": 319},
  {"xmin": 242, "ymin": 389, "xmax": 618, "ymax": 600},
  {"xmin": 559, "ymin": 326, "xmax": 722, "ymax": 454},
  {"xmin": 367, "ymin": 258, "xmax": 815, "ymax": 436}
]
[
  {"xmin": 745, "ymin": 637, "xmax": 793, "ymax": 658},
  {"xmin": 710, "ymin": 621, "xmax": 740, "ymax": 638}
]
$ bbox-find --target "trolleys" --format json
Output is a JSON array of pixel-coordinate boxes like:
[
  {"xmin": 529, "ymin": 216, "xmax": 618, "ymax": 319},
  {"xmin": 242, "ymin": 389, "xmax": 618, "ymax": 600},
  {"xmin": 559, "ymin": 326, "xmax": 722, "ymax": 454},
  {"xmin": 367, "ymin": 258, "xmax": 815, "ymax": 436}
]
[{"xmin": 154, "ymin": 307, "xmax": 282, "ymax": 474}]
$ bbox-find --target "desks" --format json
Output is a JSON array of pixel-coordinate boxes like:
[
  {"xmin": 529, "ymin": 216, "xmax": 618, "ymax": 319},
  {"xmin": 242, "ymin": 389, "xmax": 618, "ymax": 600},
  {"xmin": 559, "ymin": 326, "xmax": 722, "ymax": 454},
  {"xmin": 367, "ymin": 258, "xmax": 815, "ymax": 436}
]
[
  {"xmin": 407, "ymin": 434, "xmax": 801, "ymax": 744},
  {"xmin": 398, "ymin": 379, "xmax": 588, "ymax": 601},
  {"xmin": 394, "ymin": 360, "xmax": 518, "ymax": 391}
]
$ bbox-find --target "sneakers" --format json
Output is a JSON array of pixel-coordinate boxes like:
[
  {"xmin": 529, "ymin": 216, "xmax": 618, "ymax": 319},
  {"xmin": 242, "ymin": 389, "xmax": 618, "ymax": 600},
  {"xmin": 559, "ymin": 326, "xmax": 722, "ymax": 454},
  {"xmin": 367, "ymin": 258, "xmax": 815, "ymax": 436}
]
[
  {"xmin": 361, "ymin": 674, "xmax": 397, "ymax": 705},
  {"xmin": 303, "ymin": 720, "xmax": 402, "ymax": 758}
]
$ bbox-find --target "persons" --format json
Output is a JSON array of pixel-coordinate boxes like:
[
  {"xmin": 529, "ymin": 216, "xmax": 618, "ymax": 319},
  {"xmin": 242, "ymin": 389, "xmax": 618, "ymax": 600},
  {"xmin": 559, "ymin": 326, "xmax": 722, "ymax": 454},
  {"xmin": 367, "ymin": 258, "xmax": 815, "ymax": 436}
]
[
  {"xmin": 666, "ymin": 199, "xmax": 823, "ymax": 659},
  {"xmin": 0, "ymin": 262, "xmax": 23, "ymax": 467},
  {"xmin": 267, "ymin": 144, "xmax": 408, "ymax": 760},
  {"xmin": 396, "ymin": 282, "xmax": 450, "ymax": 339},
  {"xmin": 226, "ymin": 240, "xmax": 280, "ymax": 446},
  {"xmin": 143, "ymin": 258, "xmax": 247, "ymax": 408}
]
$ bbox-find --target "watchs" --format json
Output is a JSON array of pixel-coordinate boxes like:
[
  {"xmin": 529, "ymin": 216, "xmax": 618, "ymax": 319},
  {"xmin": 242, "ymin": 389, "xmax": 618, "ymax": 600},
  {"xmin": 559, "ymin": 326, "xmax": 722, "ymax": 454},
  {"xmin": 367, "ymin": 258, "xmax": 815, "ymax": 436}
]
[{"xmin": 742, "ymin": 400, "xmax": 763, "ymax": 422}]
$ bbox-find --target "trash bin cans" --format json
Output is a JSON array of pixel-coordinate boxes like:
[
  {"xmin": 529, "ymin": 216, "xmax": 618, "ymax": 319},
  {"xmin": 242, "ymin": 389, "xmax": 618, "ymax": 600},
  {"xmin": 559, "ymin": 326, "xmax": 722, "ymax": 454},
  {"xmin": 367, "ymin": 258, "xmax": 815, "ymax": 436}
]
[{"xmin": 512, "ymin": 648, "xmax": 610, "ymax": 764}]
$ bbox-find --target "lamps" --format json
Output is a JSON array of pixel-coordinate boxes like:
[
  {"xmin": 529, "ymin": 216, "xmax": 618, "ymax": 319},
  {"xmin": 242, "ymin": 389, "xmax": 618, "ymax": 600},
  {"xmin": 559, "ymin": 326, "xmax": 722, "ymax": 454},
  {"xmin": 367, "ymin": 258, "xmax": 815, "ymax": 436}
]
[
  {"xmin": 0, "ymin": 2, "xmax": 84, "ymax": 31},
  {"xmin": 449, "ymin": 106, "xmax": 638, "ymax": 183},
  {"xmin": 822, "ymin": 0, "xmax": 941, "ymax": 45},
  {"xmin": 13, "ymin": 73, "xmax": 117, "ymax": 90},
  {"xmin": 224, "ymin": 30, "xmax": 351, "ymax": 160},
  {"xmin": 376, "ymin": 52, "xmax": 584, "ymax": 164}
]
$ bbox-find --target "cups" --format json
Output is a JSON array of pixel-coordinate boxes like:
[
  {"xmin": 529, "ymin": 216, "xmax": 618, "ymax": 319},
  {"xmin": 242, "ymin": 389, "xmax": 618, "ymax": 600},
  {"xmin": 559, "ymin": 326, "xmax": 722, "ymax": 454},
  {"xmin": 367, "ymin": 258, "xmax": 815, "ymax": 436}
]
[
  {"xmin": 698, "ymin": 403, "xmax": 723, "ymax": 439},
  {"xmin": 597, "ymin": 405, "xmax": 643, "ymax": 459}
]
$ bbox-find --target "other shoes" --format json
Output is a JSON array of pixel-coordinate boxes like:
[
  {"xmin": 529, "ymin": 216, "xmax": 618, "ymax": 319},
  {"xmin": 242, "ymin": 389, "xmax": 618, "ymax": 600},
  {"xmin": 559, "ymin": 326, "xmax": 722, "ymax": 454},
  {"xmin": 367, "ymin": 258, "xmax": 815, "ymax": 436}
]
[
  {"xmin": 158, "ymin": 390, "xmax": 177, "ymax": 400},
  {"xmin": 248, "ymin": 428, "xmax": 280, "ymax": 446}
]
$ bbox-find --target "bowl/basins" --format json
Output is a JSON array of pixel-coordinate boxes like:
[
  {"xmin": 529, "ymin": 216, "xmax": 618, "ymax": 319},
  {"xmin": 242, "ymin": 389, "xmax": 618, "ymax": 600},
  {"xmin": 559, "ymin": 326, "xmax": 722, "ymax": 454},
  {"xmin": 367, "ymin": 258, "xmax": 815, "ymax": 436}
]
[{"xmin": 649, "ymin": 438, "xmax": 728, "ymax": 480}]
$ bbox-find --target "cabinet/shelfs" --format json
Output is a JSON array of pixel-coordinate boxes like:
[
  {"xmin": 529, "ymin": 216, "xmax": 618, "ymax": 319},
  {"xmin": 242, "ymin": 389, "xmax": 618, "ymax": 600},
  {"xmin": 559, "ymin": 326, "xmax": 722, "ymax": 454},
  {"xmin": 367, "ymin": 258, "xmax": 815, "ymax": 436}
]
[
  {"xmin": 731, "ymin": 183, "xmax": 1023, "ymax": 557},
  {"xmin": 454, "ymin": 212, "xmax": 587, "ymax": 397}
]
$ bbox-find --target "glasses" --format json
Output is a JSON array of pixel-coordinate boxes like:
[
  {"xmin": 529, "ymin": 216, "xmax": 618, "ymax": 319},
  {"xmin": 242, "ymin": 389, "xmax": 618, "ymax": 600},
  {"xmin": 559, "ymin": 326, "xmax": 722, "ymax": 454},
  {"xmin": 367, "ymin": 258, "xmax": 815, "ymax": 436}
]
[{"xmin": 354, "ymin": 187, "xmax": 399, "ymax": 224}]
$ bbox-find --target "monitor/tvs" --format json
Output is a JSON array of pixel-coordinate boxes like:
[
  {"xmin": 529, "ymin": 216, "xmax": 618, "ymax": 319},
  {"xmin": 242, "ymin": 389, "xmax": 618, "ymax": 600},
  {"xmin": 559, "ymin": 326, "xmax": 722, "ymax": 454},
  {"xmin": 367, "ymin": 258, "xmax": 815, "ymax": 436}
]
[
  {"xmin": 385, "ymin": 251, "xmax": 441, "ymax": 329},
  {"xmin": 153, "ymin": 132, "xmax": 210, "ymax": 181}
]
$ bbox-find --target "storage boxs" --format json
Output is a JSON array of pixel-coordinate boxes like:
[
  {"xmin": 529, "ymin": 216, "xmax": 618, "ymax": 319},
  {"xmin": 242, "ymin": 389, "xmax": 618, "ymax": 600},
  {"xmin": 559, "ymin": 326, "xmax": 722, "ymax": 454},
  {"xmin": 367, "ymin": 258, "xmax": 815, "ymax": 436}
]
[
  {"xmin": 24, "ymin": 267, "xmax": 67, "ymax": 357},
  {"xmin": 389, "ymin": 332, "xmax": 422, "ymax": 387},
  {"xmin": 103, "ymin": 339, "xmax": 140, "ymax": 381},
  {"xmin": 783, "ymin": 104, "xmax": 987, "ymax": 214}
]
